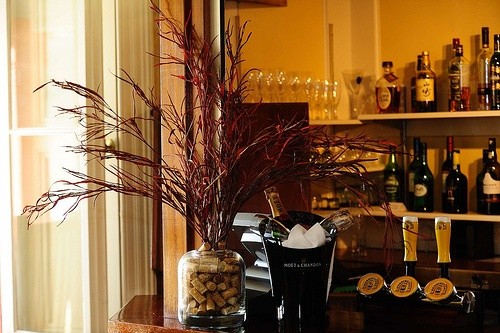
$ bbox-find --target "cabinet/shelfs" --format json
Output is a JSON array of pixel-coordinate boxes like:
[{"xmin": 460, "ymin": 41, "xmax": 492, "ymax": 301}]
[{"xmin": 309, "ymin": 110, "xmax": 500, "ymax": 289}]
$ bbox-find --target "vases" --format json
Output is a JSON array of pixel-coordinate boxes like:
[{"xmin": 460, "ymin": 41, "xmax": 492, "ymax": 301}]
[{"xmin": 177, "ymin": 248, "xmax": 246, "ymax": 331}]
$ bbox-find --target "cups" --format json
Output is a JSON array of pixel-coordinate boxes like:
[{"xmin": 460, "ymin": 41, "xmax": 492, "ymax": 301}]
[
  {"xmin": 320, "ymin": 209, "xmax": 354, "ymax": 238},
  {"xmin": 249, "ymin": 214, "xmax": 290, "ymax": 243}
]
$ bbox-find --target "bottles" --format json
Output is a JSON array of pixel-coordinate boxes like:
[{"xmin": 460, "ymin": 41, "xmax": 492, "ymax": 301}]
[
  {"xmin": 441, "ymin": 136, "xmax": 454, "ymax": 213},
  {"xmin": 476, "ymin": 27, "xmax": 492, "ymax": 111},
  {"xmin": 383, "ymin": 145, "xmax": 403, "ymax": 203},
  {"xmin": 446, "ymin": 150, "xmax": 468, "ymax": 214},
  {"xmin": 263, "ymin": 186, "xmax": 295, "ymax": 230},
  {"xmin": 407, "ymin": 137, "xmax": 420, "ymax": 212},
  {"xmin": 481, "ymin": 137, "xmax": 500, "ymax": 215},
  {"xmin": 449, "ymin": 45, "xmax": 471, "ymax": 112},
  {"xmin": 449, "ymin": 38, "xmax": 460, "ymax": 60},
  {"xmin": 416, "ymin": 51, "xmax": 437, "ymax": 112},
  {"xmin": 375, "ymin": 61, "xmax": 401, "ymax": 114},
  {"xmin": 489, "ymin": 34, "xmax": 500, "ymax": 110},
  {"xmin": 414, "ymin": 143, "xmax": 434, "ymax": 212},
  {"xmin": 476, "ymin": 148, "xmax": 489, "ymax": 214},
  {"xmin": 411, "ymin": 55, "xmax": 423, "ymax": 112}
]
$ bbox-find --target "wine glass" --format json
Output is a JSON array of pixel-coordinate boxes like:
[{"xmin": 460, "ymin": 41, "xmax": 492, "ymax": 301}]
[{"xmin": 247, "ymin": 69, "xmax": 365, "ymax": 121}]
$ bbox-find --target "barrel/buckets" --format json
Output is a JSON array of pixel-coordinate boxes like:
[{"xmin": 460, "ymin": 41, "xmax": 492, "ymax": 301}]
[{"xmin": 258, "ymin": 211, "xmax": 337, "ymax": 325}]
